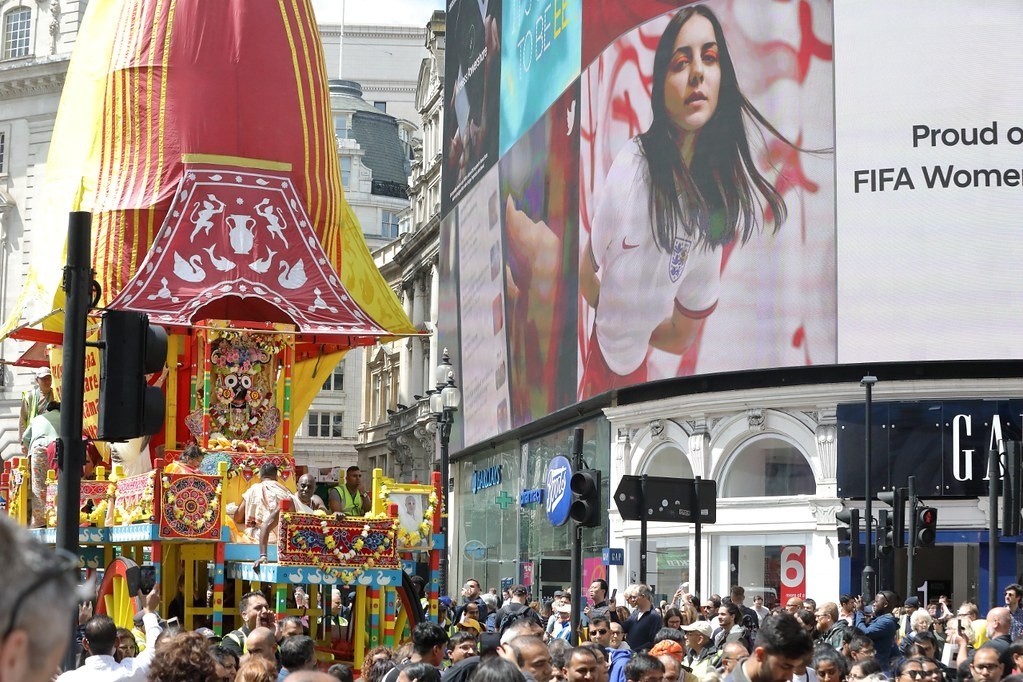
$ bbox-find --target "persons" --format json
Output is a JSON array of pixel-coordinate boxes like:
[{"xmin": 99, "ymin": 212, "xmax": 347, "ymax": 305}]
[
  {"xmin": 504, "ymin": 170, "xmax": 562, "ymax": 428},
  {"xmin": 18, "ymin": 366, "xmax": 61, "ymax": 528},
  {"xmin": 0, "ymin": 511, "xmax": 1023, "ymax": 682},
  {"xmin": 448, "ymin": 14, "xmax": 501, "ymax": 185},
  {"xmin": 163, "ymin": 446, "xmax": 205, "ymax": 474},
  {"xmin": 252, "ymin": 473, "xmax": 346, "ymax": 574},
  {"xmin": 400, "ymin": 496, "xmax": 422, "ymax": 533},
  {"xmin": 579, "ymin": 4, "xmax": 835, "ymax": 400},
  {"xmin": 328, "ymin": 466, "xmax": 371, "ymax": 516},
  {"xmin": 233, "ymin": 463, "xmax": 294, "ymax": 544}
]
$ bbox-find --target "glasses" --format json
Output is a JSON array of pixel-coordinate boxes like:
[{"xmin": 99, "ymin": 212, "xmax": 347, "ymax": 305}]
[
  {"xmin": 909, "ymin": 670, "xmax": 926, "ymax": 680},
  {"xmin": 1004, "ymin": 593, "xmax": 1012, "ymax": 597},
  {"xmin": 973, "ymin": 665, "xmax": 1001, "ymax": 674},
  {"xmin": 460, "ymin": 646, "xmax": 478, "ymax": 651},
  {"xmin": 590, "ymin": 629, "xmax": 607, "ymax": 636},
  {"xmin": 860, "ymin": 649, "xmax": 876, "ymax": 656}
]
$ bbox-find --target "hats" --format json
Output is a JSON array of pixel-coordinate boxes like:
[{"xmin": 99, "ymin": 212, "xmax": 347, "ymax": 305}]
[
  {"xmin": 905, "ymin": 597, "xmax": 918, "ymax": 609},
  {"xmin": 196, "ymin": 628, "xmax": 222, "ymax": 642},
  {"xmin": 680, "ymin": 621, "xmax": 712, "ymax": 637},
  {"xmin": 456, "ymin": 619, "xmax": 482, "ymax": 634},
  {"xmin": 133, "ymin": 610, "xmax": 160, "ymax": 626},
  {"xmin": 557, "ymin": 604, "xmax": 571, "ymax": 614},
  {"xmin": 553, "ymin": 591, "xmax": 562, "ymax": 598}
]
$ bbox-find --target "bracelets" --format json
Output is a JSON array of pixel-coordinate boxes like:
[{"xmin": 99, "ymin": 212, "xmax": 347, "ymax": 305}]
[
  {"xmin": 260, "ymin": 553, "xmax": 267, "ymax": 557},
  {"xmin": 672, "ymin": 601, "xmax": 676, "ymax": 604}
]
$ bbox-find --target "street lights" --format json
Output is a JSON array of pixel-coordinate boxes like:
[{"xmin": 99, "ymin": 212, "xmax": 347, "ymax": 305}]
[
  {"xmin": 426, "ymin": 346, "xmax": 461, "ymax": 598},
  {"xmin": 860, "ymin": 376, "xmax": 878, "ymax": 605}
]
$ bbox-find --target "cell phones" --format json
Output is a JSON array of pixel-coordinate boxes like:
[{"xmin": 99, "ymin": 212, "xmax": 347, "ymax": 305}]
[
  {"xmin": 936, "ymin": 603, "xmax": 943, "ymax": 617},
  {"xmin": 546, "ymin": 632, "xmax": 551, "ymax": 637},
  {"xmin": 165, "ymin": 617, "xmax": 181, "ymax": 632},
  {"xmin": 610, "ymin": 589, "xmax": 617, "ymax": 603},
  {"xmin": 957, "ymin": 619, "xmax": 962, "ymax": 636},
  {"xmin": 579, "ymin": 619, "xmax": 583, "ymax": 630},
  {"xmin": 480, "ymin": 631, "xmax": 500, "ymax": 661},
  {"xmin": 140, "ymin": 565, "xmax": 156, "ymax": 595}
]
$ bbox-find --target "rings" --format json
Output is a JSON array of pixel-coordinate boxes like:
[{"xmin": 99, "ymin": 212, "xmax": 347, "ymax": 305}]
[
  {"xmin": 23, "ymin": 450, "xmax": 25, "ymax": 452},
  {"xmin": 586, "ymin": 607, "xmax": 587, "ymax": 609},
  {"xmin": 153, "ymin": 589, "xmax": 158, "ymax": 593}
]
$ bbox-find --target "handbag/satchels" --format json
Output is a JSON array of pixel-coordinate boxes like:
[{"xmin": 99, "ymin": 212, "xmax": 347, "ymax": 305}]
[{"xmin": 941, "ymin": 636, "xmax": 960, "ymax": 670}]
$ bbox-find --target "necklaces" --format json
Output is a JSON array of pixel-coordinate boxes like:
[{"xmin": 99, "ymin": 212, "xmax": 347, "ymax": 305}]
[{"xmin": 209, "ymin": 391, "xmax": 272, "ymax": 440}]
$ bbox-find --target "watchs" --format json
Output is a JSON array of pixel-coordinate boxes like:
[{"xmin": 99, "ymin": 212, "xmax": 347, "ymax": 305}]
[{"xmin": 360, "ymin": 490, "xmax": 368, "ymax": 498}]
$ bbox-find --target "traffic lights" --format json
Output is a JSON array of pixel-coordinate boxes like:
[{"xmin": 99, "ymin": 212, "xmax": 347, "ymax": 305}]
[
  {"xmin": 877, "ymin": 510, "xmax": 892, "ymax": 558},
  {"xmin": 878, "ymin": 490, "xmax": 904, "ymax": 547},
  {"xmin": 570, "ymin": 470, "xmax": 601, "ymax": 527},
  {"xmin": 836, "ymin": 508, "xmax": 859, "ymax": 557},
  {"xmin": 100, "ymin": 309, "xmax": 169, "ymax": 439},
  {"xmin": 914, "ymin": 507, "xmax": 937, "ymax": 547}
]
